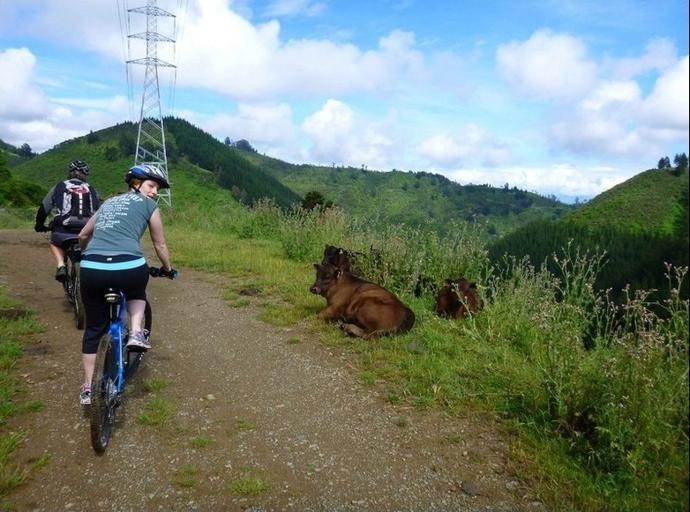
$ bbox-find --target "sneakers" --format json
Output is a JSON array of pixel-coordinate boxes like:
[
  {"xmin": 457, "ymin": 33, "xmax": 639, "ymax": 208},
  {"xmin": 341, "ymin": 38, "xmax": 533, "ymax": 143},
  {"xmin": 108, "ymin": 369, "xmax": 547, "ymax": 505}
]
[
  {"xmin": 80, "ymin": 383, "xmax": 92, "ymax": 405},
  {"xmin": 56, "ymin": 265, "xmax": 67, "ymax": 279},
  {"xmin": 127, "ymin": 329, "xmax": 152, "ymax": 349}
]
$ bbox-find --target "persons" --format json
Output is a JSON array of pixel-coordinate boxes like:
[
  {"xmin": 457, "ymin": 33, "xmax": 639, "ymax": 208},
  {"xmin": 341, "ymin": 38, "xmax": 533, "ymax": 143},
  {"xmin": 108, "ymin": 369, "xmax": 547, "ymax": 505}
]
[
  {"xmin": 34, "ymin": 159, "xmax": 106, "ymax": 280},
  {"xmin": 76, "ymin": 162, "xmax": 175, "ymax": 407}
]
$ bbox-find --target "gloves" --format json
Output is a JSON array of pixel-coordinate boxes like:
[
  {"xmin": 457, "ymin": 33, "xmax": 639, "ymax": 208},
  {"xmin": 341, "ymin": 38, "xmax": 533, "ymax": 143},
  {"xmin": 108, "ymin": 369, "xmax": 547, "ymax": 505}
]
[
  {"xmin": 35, "ymin": 222, "xmax": 46, "ymax": 231},
  {"xmin": 160, "ymin": 267, "xmax": 173, "ymax": 278}
]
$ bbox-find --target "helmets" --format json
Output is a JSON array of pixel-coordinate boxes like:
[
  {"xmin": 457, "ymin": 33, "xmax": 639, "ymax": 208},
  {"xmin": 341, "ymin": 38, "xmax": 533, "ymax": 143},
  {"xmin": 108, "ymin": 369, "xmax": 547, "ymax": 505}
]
[
  {"xmin": 126, "ymin": 164, "xmax": 169, "ymax": 189},
  {"xmin": 68, "ymin": 160, "xmax": 89, "ymax": 175}
]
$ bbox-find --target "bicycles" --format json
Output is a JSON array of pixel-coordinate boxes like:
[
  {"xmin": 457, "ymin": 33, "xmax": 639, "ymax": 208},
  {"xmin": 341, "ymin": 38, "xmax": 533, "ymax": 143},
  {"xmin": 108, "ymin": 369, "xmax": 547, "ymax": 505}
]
[
  {"xmin": 89, "ymin": 265, "xmax": 180, "ymax": 453},
  {"xmin": 40, "ymin": 226, "xmax": 85, "ymax": 331}
]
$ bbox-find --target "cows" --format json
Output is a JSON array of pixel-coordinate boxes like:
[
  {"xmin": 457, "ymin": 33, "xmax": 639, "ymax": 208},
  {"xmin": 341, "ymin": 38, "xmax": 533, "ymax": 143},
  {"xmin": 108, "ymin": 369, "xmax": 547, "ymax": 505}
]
[{"xmin": 310, "ymin": 243, "xmax": 479, "ymax": 342}]
